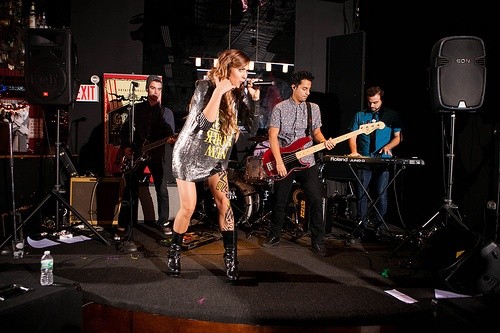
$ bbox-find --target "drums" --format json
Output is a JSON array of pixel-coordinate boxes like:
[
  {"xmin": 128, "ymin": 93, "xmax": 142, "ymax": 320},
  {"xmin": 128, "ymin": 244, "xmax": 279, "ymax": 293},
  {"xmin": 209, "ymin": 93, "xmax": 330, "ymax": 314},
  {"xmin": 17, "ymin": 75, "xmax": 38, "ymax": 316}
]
[
  {"xmin": 245, "ymin": 156, "xmax": 275, "ymax": 185},
  {"xmin": 203, "ymin": 181, "xmax": 260, "ymax": 227}
]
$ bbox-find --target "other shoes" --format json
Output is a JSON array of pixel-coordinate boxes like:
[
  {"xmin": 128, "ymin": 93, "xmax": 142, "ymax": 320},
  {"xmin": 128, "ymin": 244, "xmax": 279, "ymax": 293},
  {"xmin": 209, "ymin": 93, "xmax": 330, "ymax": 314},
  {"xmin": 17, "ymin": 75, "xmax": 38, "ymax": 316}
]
[
  {"xmin": 262, "ymin": 236, "xmax": 280, "ymax": 247},
  {"xmin": 158, "ymin": 224, "xmax": 172, "ymax": 238},
  {"xmin": 356, "ymin": 225, "xmax": 368, "ymax": 239},
  {"xmin": 117, "ymin": 234, "xmax": 137, "ymax": 253},
  {"xmin": 310, "ymin": 240, "xmax": 326, "ymax": 256},
  {"xmin": 376, "ymin": 225, "xmax": 383, "ymax": 236}
]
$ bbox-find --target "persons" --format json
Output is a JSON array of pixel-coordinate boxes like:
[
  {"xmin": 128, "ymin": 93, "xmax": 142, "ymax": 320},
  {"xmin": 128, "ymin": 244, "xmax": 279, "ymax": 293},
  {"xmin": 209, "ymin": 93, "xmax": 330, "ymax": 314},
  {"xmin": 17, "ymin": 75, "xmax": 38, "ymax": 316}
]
[
  {"xmin": 114, "ymin": 76, "xmax": 177, "ymax": 240},
  {"xmin": 347, "ymin": 86, "xmax": 401, "ymax": 239},
  {"xmin": 265, "ymin": 70, "xmax": 336, "ymax": 256},
  {"xmin": 167, "ymin": 50, "xmax": 263, "ymax": 281}
]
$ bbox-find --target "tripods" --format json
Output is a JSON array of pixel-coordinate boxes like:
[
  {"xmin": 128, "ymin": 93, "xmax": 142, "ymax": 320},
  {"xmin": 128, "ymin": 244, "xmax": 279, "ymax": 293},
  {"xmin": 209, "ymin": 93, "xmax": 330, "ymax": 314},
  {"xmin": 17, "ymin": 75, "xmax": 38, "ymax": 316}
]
[
  {"xmin": 0, "ymin": 108, "xmax": 112, "ymax": 249},
  {"xmin": 387, "ymin": 110, "xmax": 475, "ymax": 257}
]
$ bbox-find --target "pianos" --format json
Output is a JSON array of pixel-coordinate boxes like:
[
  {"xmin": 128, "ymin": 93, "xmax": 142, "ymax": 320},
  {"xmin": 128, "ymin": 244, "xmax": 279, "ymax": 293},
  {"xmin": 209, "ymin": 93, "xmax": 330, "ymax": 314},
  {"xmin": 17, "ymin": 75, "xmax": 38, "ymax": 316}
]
[{"xmin": 320, "ymin": 154, "xmax": 424, "ymax": 241}]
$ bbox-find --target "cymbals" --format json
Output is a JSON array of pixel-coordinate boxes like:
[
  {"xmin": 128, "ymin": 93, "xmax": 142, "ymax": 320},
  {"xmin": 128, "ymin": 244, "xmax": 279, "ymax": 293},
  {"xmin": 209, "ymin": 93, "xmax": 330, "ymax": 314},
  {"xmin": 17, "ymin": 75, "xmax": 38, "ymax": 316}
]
[{"xmin": 248, "ymin": 135, "xmax": 269, "ymax": 141}]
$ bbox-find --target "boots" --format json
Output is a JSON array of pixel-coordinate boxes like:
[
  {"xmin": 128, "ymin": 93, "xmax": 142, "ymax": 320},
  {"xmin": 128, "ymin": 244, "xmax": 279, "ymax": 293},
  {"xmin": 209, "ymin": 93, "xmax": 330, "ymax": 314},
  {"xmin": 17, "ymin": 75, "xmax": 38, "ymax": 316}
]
[
  {"xmin": 221, "ymin": 229, "xmax": 238, "ymax": 280},
  {"xmin": 167, "ymin": 229, "xmax": 186, "ymax": 276}
]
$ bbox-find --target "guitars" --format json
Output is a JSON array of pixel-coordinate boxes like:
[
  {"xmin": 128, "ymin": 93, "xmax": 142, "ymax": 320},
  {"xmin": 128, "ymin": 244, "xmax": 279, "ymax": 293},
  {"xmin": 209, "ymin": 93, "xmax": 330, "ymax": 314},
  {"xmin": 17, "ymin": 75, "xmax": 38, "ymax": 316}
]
[
  {"xmin": 262, "ymin": 119, "xmax": 386, "ymax": 181},
  {"xmin": 116, "ymin": 130, "xmax": 179, "ymax": 172}
]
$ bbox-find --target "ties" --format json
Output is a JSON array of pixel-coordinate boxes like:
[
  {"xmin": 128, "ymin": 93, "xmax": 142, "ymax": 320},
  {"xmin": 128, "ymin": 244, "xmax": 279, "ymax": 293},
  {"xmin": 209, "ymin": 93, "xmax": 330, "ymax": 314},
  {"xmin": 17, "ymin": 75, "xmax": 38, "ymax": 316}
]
[{"xmin": 369, "ymin": 113, "xmax": 376, "ymax": 155}]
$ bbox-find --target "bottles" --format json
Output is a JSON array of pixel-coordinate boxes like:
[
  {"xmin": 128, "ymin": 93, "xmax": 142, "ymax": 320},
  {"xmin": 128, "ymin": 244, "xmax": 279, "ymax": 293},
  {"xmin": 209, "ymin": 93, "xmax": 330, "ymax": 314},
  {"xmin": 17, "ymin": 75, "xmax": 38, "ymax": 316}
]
[{"xmin": 40, "ymin": 250, "xmax": 54, "ymax": 286}]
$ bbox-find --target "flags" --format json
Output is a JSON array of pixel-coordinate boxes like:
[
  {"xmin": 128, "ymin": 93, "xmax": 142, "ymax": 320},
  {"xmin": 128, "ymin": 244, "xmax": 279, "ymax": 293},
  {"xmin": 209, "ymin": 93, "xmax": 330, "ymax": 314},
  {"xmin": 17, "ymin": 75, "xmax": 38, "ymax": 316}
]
[
  {"xmin": 241, "ymin": 0, "xmax": 248, "ymax": 12},
  {"xmin": 259, "ymin": 0, "xmax": 269, "ymax": 21}
]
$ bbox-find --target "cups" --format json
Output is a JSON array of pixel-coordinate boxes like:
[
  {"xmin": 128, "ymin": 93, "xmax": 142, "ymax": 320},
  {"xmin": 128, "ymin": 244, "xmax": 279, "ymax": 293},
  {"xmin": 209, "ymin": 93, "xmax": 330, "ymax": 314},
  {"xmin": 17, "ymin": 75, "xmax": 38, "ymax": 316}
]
[{"xmin": 12, "ymin": 239, "xmax": 25, "ymax": 259}]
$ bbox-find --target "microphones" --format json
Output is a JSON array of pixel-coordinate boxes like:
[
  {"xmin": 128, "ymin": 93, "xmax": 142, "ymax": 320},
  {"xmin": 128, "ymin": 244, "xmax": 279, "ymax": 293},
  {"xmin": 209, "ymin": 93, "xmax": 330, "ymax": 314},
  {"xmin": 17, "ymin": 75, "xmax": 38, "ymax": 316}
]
[
  {"xmin": 243, "ymin": 80, "xmax": 275, "ymax": 87},
  {"xmin": 131, "ymin": 81, "xmax": 141, "ymax": 87}
]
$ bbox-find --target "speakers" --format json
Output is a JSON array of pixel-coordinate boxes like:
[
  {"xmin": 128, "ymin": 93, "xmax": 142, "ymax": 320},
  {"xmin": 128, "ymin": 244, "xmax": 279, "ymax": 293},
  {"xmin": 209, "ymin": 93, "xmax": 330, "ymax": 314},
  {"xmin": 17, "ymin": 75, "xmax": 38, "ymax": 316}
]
[
  {"xmin": 23, "ymin": 27, "xmax": 74, "ymax": 107},
  {"xmin": 67, "ymin": 178, "xmax": 126, "ymax": 225},
  {"xmin": 431, "ymin": 35, "xmax": 488, "ymax": 111},
  {"xmin": 443, "ymin": 241, "xmax": 500, "ymax": 301}
]
